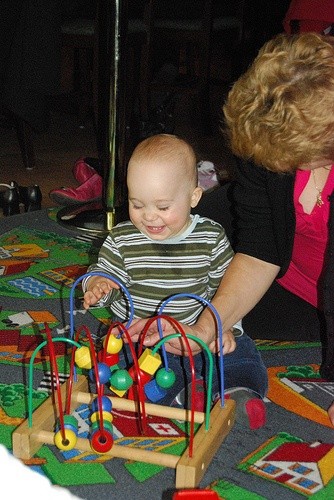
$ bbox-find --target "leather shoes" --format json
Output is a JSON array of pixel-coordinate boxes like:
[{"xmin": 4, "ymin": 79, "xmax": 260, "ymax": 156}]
[
  {"xmin": 0, "ymin": 188, "xmax": 20, "ymax": 216},
  {"xmin": 20, "ymin": 186, "xmax": 42, "ymax": 212}
]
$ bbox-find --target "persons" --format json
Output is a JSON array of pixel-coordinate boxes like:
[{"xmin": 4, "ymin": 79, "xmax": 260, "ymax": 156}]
[
  {"xmin": 82, "ymin": 134, "xmax": 269, "ymax": 428},
  {"xmin": 110, "ymin": 32, "xmax": 334, "ymax": 426}
]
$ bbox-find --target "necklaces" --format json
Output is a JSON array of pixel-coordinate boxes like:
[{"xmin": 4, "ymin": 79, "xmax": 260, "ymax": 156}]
[{"xmin": 312, "ymin": 171, "xmax": 325, "ymax": 210}]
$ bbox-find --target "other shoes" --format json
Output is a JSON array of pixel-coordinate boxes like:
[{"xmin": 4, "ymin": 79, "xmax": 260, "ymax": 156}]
[
  {"xmin": 49, "ymin": 174, "xmax": 103, "ymax": 205},
  {"xmin": 73, "ymin": 163, "xmax": 96, "ymax": 185}
]
